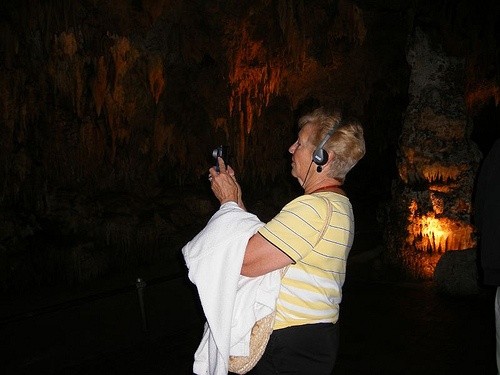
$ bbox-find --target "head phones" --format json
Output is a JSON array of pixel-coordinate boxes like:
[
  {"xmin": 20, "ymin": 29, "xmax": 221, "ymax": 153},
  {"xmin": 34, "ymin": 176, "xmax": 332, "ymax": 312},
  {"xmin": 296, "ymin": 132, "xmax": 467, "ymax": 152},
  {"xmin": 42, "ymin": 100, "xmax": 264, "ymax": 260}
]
[{"xmin": 313, "ymin": 117, "xmax": 346, "ymax": 166}]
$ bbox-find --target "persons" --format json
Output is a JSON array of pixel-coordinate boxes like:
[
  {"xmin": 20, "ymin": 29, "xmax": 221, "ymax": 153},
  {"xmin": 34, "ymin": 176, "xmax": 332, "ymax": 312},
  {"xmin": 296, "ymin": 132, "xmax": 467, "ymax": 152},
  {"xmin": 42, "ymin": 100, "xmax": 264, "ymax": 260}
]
[{"xmin": 209, "ymin": 108, "xmax": 367, "ymax": 375}]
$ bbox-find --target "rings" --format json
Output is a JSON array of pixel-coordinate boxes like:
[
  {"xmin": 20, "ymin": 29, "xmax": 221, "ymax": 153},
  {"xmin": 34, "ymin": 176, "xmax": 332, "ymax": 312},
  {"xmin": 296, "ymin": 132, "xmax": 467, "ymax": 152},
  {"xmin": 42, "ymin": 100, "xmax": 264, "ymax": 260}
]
[{"xmin": 209, "ymin": 176, "xmax": 213, "ymax": 182}]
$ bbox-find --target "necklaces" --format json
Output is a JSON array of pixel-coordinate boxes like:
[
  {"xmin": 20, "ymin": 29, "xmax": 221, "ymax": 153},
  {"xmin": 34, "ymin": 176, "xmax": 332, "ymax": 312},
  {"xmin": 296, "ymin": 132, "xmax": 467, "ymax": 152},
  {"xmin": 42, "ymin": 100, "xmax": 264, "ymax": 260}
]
[{"xmin": 309, "ymin": 185, "xmax": 345, "ymax": 195}]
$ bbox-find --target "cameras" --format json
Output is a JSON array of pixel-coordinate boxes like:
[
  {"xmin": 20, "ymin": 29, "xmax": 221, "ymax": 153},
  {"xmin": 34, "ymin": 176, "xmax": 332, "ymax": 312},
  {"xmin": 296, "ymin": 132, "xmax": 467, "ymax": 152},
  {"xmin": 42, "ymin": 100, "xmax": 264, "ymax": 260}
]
[{"xmin": 212, "ymin": 145, "xmax": 229, "ymax": 173}]
